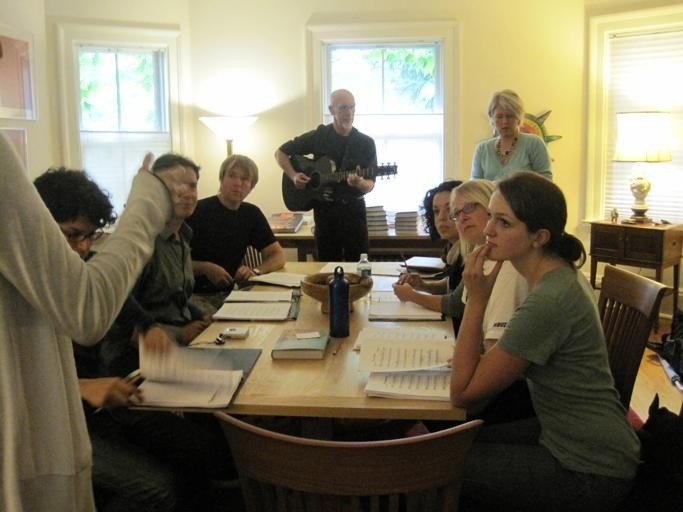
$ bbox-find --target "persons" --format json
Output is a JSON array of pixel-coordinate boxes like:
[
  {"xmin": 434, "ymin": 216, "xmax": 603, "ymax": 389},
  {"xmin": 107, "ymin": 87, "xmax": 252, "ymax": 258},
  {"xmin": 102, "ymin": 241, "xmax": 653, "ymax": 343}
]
[
  {"xmin": 34, "ymin": 164, "xmax": 176, "ymax": 511},
  {"xmin": 274, "ymin": 87, "xmax": 379, "ymax": 261},
  {"xmin": 0, "ymin": 107, "xmax": 177, "ymax": 512},
  {"xmin": 95, "ymin": 153, "xmax": 213, "ymax": 386},
  {"xmin": 186, "ymin": 153, "xmax": 287, "ymax": 290},
  {"xmin": 449, "ymin": 170, "xmax": 643, "ymax": 512},
  {"xmin": 470, "ymin": 89, "xmax": 553, "ymax": 182},
  {"xmin": 393, "ymin": 180, "xmax": 465, "ymax": 319},
  {"xmin": 447, "ymin": 179, "xmax": 529, "ymax": 368}
]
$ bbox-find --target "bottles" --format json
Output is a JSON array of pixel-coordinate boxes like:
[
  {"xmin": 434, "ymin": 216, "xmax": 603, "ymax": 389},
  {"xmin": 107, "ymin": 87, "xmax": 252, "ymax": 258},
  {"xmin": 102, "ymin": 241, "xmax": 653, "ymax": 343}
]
[
  {"xmin": 354, "ymin": 253, "xmax": 371, "ymax": 306},
  {"xmin": 328, "ymin": 266, "xmax": 351, "ymax": 337}
]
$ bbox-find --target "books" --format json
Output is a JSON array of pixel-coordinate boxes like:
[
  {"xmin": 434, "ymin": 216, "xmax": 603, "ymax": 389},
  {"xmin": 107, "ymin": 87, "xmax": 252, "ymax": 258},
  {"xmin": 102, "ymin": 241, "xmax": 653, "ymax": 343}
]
[
  {"xmin": 271, "ymin": 328, "xmax": 331, "ymax": 360},
  {"xmin": 267, "ymin": 212, "xmax": 304, "ymax": 232},
  {"xmin": 366, "ymin": 205, "xmax": 418, "ymax": 232}
]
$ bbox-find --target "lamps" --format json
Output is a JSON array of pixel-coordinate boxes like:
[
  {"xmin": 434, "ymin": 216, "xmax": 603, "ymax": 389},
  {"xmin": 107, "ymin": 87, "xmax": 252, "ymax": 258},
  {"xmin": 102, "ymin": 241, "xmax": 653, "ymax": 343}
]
[
  {"xmin": 610, "ymin": 111, "xmax": 679, "ymax": 223},
  {"xmin": 194, "ymin": 113, "xmax": 254, "ymax": 156}
]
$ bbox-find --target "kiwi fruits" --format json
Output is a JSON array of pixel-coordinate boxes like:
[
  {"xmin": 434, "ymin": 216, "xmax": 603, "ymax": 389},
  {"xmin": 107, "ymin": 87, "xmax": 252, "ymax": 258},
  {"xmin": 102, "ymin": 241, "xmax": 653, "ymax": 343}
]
[{"xmin": 327, "ymin": 274, "xmax": 350, "ymax": 284}]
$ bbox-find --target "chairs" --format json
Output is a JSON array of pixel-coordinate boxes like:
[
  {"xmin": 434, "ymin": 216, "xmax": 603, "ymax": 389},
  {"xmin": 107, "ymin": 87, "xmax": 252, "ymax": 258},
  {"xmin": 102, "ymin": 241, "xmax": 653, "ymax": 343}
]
[
  {"xmin": 594, "ymin": 264, "xmax": 673, "ymax": 412},
  {"xmin": 215, "ymin": 407, "xmax": 485, "ymax": 510}
]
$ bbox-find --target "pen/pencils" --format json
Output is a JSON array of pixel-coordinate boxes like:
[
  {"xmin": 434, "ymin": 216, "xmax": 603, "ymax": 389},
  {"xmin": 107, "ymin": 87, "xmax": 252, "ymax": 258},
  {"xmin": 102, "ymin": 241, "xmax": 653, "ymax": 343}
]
[
  {"xmin": 402, "ymin": 255, "xmax": 411, "ymax": 274},
  {"xmin": 333, "ymin": 342, "xmax": 341, "ymax": 354},
  {"xmin": 91, "ymin": 375, "xmax": 146, "ymax": 416}
]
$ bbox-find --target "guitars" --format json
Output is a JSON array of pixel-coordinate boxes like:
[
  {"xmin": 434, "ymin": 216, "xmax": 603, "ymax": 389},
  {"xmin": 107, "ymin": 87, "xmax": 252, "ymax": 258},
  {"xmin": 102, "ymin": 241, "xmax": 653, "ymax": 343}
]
[{"xmin": 282, "ymin": 155, "xmax": 398, "ymax": 212}]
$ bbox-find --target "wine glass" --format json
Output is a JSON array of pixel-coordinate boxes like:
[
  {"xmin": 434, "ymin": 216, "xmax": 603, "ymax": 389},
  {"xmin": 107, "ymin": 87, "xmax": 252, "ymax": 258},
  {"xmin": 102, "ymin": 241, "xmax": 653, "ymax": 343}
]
[{"xmin": 301, "ymin": 271, "xmax": 373, "ymax": 315}]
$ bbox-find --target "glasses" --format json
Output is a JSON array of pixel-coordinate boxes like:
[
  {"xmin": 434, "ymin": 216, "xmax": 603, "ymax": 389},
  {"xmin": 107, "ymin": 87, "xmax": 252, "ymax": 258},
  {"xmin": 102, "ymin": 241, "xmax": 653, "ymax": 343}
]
[
  {"xmin": 450, "ymin": 203, "xmax": 480, "ymax": 220},
  {"xmin": 62, "ymin": 225, "xmax": 103, "ymax": 242}
]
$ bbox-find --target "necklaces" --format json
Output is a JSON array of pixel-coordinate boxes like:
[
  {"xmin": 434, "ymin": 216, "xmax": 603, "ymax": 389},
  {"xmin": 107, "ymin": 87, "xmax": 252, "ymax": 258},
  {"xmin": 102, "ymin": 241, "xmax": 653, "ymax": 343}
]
[{"xmin": 495, "ymin": 136, "xmax": 518, "ymax": 157}]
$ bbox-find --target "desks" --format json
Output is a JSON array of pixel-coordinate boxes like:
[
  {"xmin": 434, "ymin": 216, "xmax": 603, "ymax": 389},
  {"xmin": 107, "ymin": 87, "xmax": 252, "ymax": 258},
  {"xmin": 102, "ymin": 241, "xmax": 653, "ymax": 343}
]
[
  {"xmin": 264, "ymin": 227, "xmax": 448, "ymax": 261},
  {"xmin": 588, "ymin": 218, "xmax": 680, "ymax": 330},
  {"xmin": 120, "ymin": 258, "xmax": 457, "ymax": 451}
]
